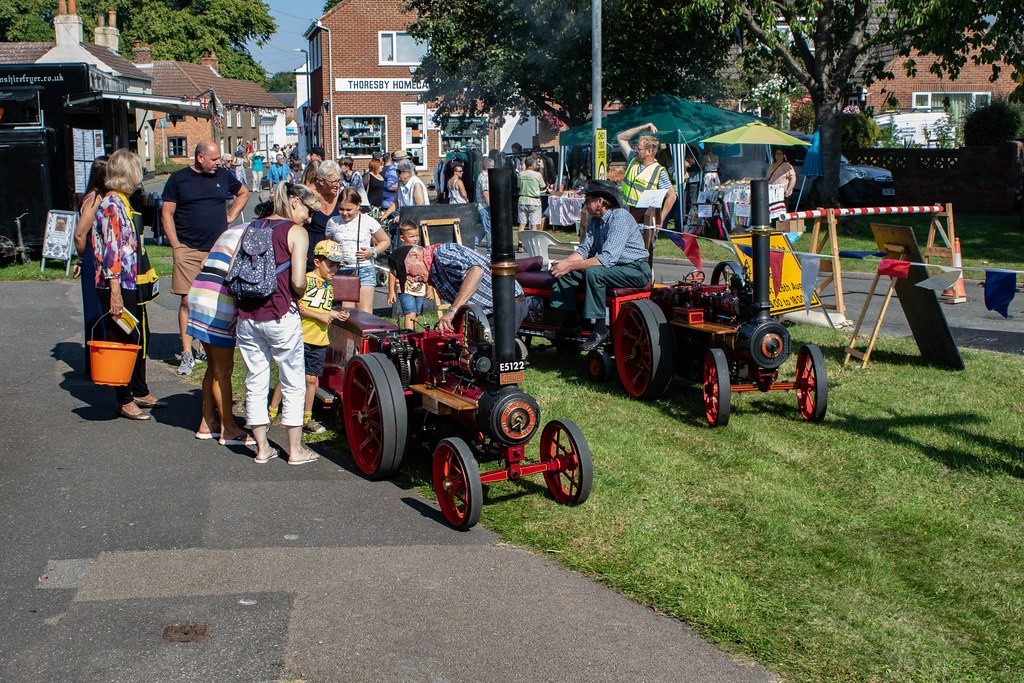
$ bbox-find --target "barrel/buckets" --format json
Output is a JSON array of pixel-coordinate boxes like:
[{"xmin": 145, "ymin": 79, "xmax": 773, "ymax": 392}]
[{"xmin": 87, "ymin": 312, "xmax": 143, "ymax": 387}]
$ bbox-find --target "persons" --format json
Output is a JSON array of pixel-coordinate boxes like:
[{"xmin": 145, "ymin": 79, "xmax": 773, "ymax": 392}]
[
  {"xmin": 74, "ymin": 148, "xmax": 171, "ymax": 420},
  {"xmin": 220, "ymin": 140, "xmax": 298, "ymax": 215},
  {"xmin": 186, "ymin": 181, "xmax": 350, "ymax": 466},
  {"xmin": 326, "ymin": 187, "xmax": 392, "ymax": 314},
  {"xmin": 302, "ymin": 160, "xmax": 346, "ymax": 272},
  {"xmin": 267, "ymin": 147, "xmax": 326, "ymax": 200},
  {"xmin": 447, "ymin": 164, "xmax": 469, "ymax": 205},
  {"xmin": 664, "ymin": 154, "xmax": 696, "ymax": 231},
  {"xmin": 339, "ymin": 150, "xmax": 430, "ymax": 248},
  {"xmin": 700, "ymin": 146, "xmax": 721, "ymax": 189},
  {"xmin": 389, "ymin": 220, "xmax": 531, "ymax": 346},
  {"xmin": 473, "ymin": 158, "xmax": 495, "ymax": 257},
  {"xmin": 515, "ymin": 157, "xmax": 546, "ymax": 254},
  {"xmin": 619, "ymin": 122, "xmax": 679, "ymax": 270},
  {"xmin": 511, "ymin": 142, "xmax": 523, "ymax": 154},
  {"xmin": 770, "ymin": 147, "xmax": 797, "ymax": 211},
  {"xmin": 162, "ymin": 141, "xmax": 251, "ymax": 377},
  {"xmin": 546, "ymin": 177, "xmax": 653, "ymax": 351}
]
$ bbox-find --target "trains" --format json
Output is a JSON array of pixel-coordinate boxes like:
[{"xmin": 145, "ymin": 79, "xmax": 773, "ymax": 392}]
[
  {"xmin": 516, "ymin": 120, "xmax": 829, "ymax": 426},
  {"xmin": 320, "ymin": 150, "xmax": 594, "ymax": 531}
]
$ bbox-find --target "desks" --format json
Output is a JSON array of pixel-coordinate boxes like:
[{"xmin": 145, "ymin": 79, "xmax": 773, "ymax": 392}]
[{"xmin": 547, "ymin": 195, "xmax": 586, "ymax": 237}]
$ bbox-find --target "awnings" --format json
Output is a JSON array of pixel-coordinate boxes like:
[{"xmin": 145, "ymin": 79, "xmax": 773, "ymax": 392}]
[{"xmin": 62, "ymin": 88, "xmax": 215, "ymax": 120}]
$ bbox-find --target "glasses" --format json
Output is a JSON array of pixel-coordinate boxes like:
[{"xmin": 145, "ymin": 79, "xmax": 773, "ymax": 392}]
[
  {"xmin": 634, "ymin": 146, "xmax": 645, "ymax": 153},
  {"xmin": 226, "ymin": 160, "xmax": 233, "ymax": 163},
  {"xmin": 456, "ymin": 170, "xmax": 463, "ymax": 173},
  {"xmin": 295, "ymin": 194, "xmax": 315, "ymax": 218},
  {"xmin": 397, "ymin": 169, "xmax": 402, "ymax": 174},
  {"xmin": 323, "ymin": 177, "xmax": 341, "ymax": 187}
]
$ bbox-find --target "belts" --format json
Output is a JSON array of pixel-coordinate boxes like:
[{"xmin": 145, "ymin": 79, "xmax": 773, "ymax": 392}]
[{"xmin": 515, "ymin": 295, "xmax": 526, "ymax": 303}]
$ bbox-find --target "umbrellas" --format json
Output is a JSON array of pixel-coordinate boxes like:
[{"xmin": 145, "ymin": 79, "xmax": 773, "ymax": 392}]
[
  {"xmin": 794, "ymin": 125, "xmax": 822, "ymax": 213},
  {"xmin": 699, "ymin": 120, "xmax": 813, "ymax": 178}
]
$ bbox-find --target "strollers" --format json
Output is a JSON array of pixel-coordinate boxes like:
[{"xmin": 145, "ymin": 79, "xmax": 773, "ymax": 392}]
[{"xmin": 371, "ymin": 217, "xmax": 398, "ymax": 288}]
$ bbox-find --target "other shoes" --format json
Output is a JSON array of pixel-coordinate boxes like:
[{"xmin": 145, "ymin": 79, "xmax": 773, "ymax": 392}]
[
  {"xmin": 132, "ymin": 396, "xmax": 168, "ymax": 407},
  {"xmin": 117, "ymin": 404, "xmax": 151, "ymax": 419},
  {"xmin": 517, "ymin": 246, "xmax": 524, "ymax": 252},
  {"xmin": 543, "ymin": 323, "xmax": 582, "ymax": 340},
  {"xmin": 583, "ymin": 327, "xmax": 609, "ymax": 351}
]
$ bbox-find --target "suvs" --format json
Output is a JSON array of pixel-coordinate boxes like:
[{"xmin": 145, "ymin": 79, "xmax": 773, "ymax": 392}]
[{"xmin": 714, "ymin": 131, "xmax": 896, "ymax": 206}]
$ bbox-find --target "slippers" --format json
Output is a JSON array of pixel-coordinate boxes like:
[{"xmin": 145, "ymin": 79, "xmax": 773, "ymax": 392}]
[
  {"xmin": 218, "ymin": 431, "xmax": 257, "ymax": 446},
  {"xmin": 253, "ymin": 446, "xmax": 280, "ymax": 464},
  {"xmin": 195, "ymin": 431, "xmax": 220, "ymax": 440},
  {"xmin": 288, "ymin": 448, "xmax": 319, "ymax": 465}
]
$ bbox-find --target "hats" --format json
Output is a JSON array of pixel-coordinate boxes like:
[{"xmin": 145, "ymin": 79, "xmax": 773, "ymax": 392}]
[
  {"xmin": 392, "ymin": 150, "xmax": 411, "ymax": 160},
  {"xmin": 387, "ymin": 245, "xmax": 413, "ymax": 294},
  {"xmin": 373, "ymin": 153, "xmax": 381, "ymax": 159},
  {"xmin": 577, "ymin": 179, "xmax": 622, "ymax": 208},
  {"xmin": 236, "ymin": 150, "xmax": 244, "ymax": 157},
  {"xmin": 313, "ymin": 239, "xmax": 344, "ymax": 263},
  {"xmin": 397, "ymin": 162, "xmax": 411, "ymax": 172},
  {"xmin": 306, "ymin": 147, "xmax": 325, "ymax": 157}
]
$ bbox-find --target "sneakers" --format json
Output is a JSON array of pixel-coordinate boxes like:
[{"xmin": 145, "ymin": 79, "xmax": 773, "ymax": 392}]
[
  {"xmin": 194, "ymin": 351, "xmax": 208, "ymax": 364},
  {"xmin": 176, "ymin": 351, "xmax": 195, "ymax": 375},
  {"xmin": 266, "ymin": 413, "xmax": 278, "ymax": 433},
  {"xmin": 302, "ymin": 420, "xmax": 326, "ymax": 434}
]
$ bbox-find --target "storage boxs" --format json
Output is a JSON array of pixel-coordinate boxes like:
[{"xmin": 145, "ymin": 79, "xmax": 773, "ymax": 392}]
[{"xmin": 777, "ymin": 220, "xmax": 805, "ymax": 232}]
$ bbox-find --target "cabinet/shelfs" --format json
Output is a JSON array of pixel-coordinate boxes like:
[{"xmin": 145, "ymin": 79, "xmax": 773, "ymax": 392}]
[
  {"xmin": 339, "ymin": 127, "xmax": 386, "ymax": 149},
  {"xmin": 442, "ymin": 121, "xmax": 482, "ymax": 150}
]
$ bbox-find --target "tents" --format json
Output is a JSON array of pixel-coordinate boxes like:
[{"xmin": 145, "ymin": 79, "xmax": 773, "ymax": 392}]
[{"xmin": 555, "ymin": 94, "xmax": 775, "ymax": 233}]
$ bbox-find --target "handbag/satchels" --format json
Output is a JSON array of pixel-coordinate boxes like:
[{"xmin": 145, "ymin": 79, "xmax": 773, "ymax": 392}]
[{"xmin": 330, "ymin": 275, "xmax": 361, "ymax": 302}]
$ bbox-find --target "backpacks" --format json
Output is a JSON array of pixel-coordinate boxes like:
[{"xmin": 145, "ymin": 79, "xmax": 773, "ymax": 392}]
[{"xmin": 224, "ymin": 219, "xmax": 292, "ymax": 303}]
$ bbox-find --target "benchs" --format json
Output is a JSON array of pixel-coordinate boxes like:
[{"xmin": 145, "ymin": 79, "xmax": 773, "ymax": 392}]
[
  {"xmin": 333, "ymin": 276, "xmax": 396, "ymax": 336},
  {"xmin": 515, "ymin": 256, "xmax": 652, "ymax": 335}
]
